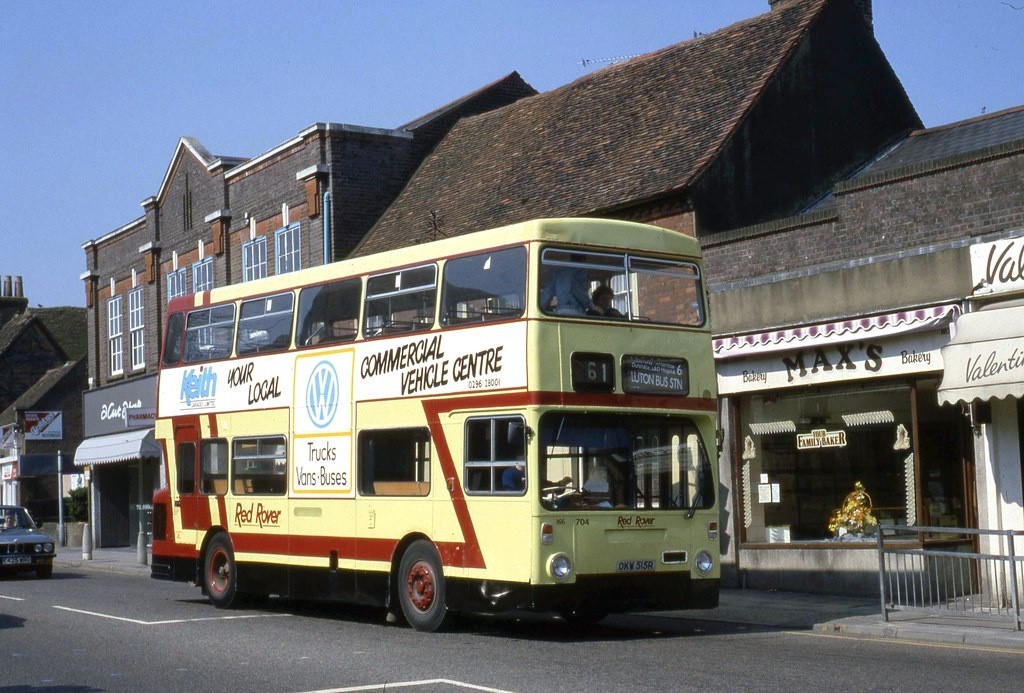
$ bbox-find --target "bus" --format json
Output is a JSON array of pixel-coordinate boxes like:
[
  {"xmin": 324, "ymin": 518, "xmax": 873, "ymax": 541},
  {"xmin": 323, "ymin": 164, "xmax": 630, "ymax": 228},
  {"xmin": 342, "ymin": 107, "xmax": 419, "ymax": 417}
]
[{"xmin": 151, "ymin": 217, "xmax": 721, "ymax": 632}]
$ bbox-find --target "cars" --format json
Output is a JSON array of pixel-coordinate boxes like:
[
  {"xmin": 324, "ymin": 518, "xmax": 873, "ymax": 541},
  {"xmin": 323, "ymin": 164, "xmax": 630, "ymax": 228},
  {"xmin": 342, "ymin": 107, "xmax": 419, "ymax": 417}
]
[{"xmin": 0, "ymin": 505, "xmax": 56, "ymax": 579}]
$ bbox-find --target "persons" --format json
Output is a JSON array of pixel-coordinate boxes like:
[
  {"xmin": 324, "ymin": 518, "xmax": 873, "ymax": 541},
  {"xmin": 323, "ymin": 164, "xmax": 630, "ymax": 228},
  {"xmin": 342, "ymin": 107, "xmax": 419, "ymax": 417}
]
[
  {"xmin": 540, "ymin": 248, "xmax": 634, "ymax": 321},
  {"xmin": 503, "ymin": 447, "xmax": 576, "ymax": 497},
  {"xmin": 0, "ymin": 509, "xmax": 20, "ymax": 531}
]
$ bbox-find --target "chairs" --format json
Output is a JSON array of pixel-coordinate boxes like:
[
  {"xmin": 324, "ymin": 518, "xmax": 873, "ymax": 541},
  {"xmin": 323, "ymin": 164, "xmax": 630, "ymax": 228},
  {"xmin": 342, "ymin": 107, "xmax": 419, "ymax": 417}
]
[
  {"xmin": 207, "ymin": 307, "xmax": 522, "ymax": 357},
  {"xmin": 377, "ymin": 473, "xmax": 400, "ymax": 480}
]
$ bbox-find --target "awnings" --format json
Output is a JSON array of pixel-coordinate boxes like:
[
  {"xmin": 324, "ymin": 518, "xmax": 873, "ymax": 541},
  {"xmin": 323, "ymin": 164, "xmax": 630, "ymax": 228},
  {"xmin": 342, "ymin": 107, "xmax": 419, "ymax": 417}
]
[
  {"xmin": 936, "ymin": 298, "xmax": 1024, "ymax": 407},
  {"xmin": 73, "ymin": 427, "xmax": 164, "ymax": 466},
  {"xmin": 712, "ymin": 304, "xmax": 963, "ymax": 359}
]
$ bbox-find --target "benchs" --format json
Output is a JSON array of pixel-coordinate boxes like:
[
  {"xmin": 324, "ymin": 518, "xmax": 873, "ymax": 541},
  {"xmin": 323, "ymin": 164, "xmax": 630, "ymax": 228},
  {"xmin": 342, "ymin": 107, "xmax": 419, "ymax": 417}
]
[
  {"xmin": 210, "ymin": 477, "xmax": 254, "ymax": 493},
  {"xmin": 373, "ymin": 481, "xmax": 430, "ymax": 496}
]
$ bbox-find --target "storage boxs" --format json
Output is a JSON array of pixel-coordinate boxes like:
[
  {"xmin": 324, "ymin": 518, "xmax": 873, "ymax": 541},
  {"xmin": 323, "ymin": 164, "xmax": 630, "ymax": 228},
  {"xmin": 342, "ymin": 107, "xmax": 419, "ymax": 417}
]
[{"xmin": 764, "ymin": 524, "xmax": 791, "ymax": 543}]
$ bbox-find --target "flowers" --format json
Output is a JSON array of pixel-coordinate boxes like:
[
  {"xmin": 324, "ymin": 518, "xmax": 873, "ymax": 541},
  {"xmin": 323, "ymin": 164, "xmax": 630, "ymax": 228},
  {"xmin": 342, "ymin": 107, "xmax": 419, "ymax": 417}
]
[{"xmin": 829, "ymin": 481, "xmax": 877, "ymax": 535}]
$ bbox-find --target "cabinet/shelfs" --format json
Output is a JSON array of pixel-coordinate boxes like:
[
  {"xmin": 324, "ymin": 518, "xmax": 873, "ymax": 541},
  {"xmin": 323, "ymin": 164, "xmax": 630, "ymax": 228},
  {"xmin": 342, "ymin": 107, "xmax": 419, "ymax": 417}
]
[{"xmin": 763, "ymin": 441, "xmax": 871, "ymax": 526}]
